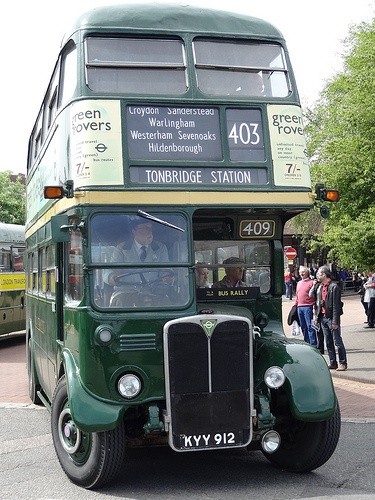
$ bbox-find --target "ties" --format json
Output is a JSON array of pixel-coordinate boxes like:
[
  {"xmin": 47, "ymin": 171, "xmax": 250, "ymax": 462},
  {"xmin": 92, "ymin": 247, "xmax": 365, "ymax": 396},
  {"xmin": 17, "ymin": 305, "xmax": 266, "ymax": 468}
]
[{"xmin": 139, "ymin": 246, "xmax": 147, "ymax": 262}]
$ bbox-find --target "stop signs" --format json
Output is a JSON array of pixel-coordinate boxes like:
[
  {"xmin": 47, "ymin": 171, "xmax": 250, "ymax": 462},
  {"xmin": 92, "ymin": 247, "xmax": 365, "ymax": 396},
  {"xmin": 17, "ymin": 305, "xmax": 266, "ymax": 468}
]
[{"xmin": 285, "ymin": 248, "xmax": 297, "ymax": 260}]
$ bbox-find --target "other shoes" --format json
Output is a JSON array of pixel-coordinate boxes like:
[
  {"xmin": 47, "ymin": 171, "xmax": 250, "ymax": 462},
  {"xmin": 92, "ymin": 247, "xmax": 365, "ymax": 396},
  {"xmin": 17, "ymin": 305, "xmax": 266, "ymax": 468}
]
[
  {"xmin": 328, "ymin": 363, "xmax": 338, "ymax": 369},
  {"xmin": 336, "ymin": 363, "xmax": 347, "ymax": 371}
]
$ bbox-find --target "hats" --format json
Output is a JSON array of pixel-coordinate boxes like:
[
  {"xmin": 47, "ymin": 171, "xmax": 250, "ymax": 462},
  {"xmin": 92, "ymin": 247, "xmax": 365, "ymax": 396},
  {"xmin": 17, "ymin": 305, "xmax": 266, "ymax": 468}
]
[{"xmin": 128, "ymin": 215, "xmax": 150, "ymax": 227}]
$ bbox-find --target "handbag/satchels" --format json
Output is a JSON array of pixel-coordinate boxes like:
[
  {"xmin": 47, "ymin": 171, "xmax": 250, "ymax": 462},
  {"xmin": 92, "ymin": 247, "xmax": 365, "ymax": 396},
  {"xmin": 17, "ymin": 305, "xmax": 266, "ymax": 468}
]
[{"xmin": 325, "ymin": 302, "xmax": 344, "ymax": 318}]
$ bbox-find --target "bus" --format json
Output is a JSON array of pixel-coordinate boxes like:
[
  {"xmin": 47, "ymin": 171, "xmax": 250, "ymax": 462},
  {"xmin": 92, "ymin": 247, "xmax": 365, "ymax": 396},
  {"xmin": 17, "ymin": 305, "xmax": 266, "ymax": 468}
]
[
  {"xmin": 24, "ymin": 1, "xmax": 342, "ymax": 492},
  {"xmin": 1, "ymin": 223, "xmax": 25, "ymax": 344}
]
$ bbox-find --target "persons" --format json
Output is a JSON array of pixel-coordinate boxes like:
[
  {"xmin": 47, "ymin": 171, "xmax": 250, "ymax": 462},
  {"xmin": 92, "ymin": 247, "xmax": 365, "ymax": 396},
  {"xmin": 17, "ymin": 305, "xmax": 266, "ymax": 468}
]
[
  {"xmin": 314, "ymin": 266, "xmax": 348, "ymax": 372},
  {"xmin": 308, "ymin": 270, "xmax": 330, "ymax": 355},
  {"xmin": 194, "ymin": 261, "xmax": 212, "ymax": 288},
  {"xmin": 247, "ymin": 261, "xmax": 375, "ymax": 330},
  {"xmin": 296, "ymin": 265, "xmax": 317, "ymax": 347},
  {"xmin": 211, "ymin": 257, "xmax": 250, "ymax": 288},
  {"xmin": 103, "ymin": 216, "xmax": 175, "ymax": 307}
]
